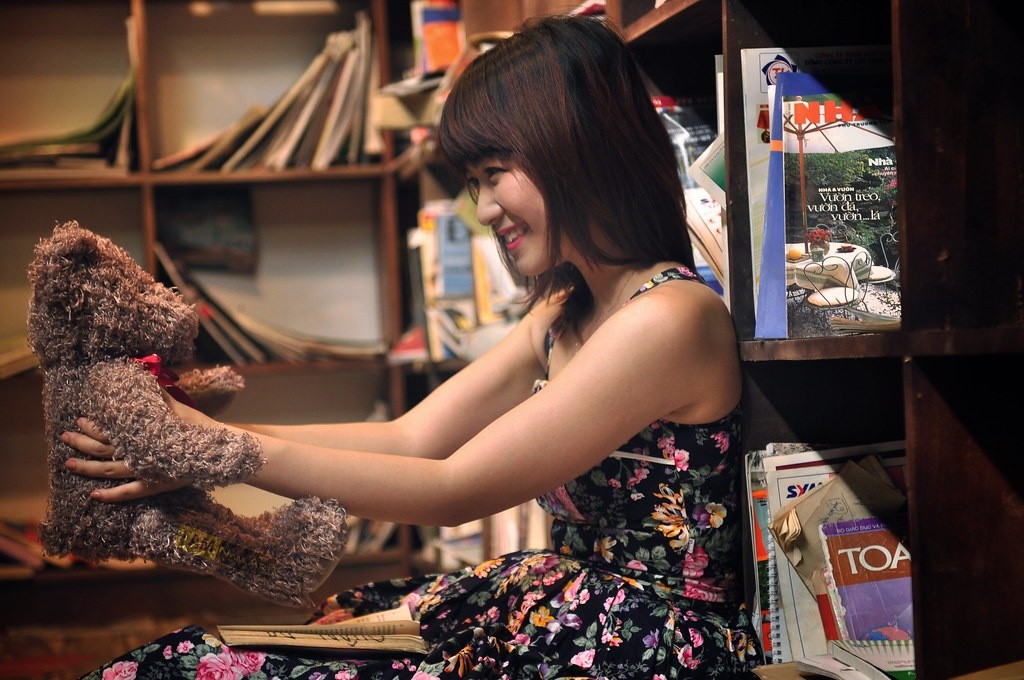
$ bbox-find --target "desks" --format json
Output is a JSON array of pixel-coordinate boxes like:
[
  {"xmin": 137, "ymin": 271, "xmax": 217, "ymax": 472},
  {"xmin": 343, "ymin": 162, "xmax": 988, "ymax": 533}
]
[{"xmin": 786, "ymin": 242, "xmax": 872, "ymax": 336}]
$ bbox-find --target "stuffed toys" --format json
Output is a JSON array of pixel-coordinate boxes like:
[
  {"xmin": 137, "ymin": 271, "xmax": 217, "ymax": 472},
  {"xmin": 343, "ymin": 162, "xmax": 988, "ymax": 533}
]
[{"xmin": 25, "ymin": 221, "xmax": 349, "ymax": 609}]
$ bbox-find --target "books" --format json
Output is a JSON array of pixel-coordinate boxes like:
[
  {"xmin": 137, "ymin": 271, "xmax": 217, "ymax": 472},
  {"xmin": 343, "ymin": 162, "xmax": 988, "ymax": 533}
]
[
  {"xmin": 682, "ymin": 48, "xmax": 901, "ymax": 340},
  {"xmin": 0, "ymin": 64, "xmax": 135, "ymax": 172},
  {"xmin": 156, "ymin": 19, "xmax": 388, "ymax": 171},
  {"xmin": 153, "ymin": 241, "xmax": 376, "ymax": 365},
  {"xmin": 217, "ymin": 600, "xmax": 427, "ymax": 656},
  {"xmin": 744, "ymin": 441, "xmax": 916, "ymax": 680}
]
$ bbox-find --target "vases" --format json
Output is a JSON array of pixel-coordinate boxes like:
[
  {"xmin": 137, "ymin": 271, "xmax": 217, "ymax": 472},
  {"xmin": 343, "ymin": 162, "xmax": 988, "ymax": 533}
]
[{"xmin": 809, "ymin": 241, "xmax": 830, "ymax": 256}]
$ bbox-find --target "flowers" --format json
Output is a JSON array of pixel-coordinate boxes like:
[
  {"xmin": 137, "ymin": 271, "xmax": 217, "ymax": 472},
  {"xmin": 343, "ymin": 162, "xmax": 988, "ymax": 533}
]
[{"xmin": 806, "ymin": 229, "xmax": 832, "ymax": 242}]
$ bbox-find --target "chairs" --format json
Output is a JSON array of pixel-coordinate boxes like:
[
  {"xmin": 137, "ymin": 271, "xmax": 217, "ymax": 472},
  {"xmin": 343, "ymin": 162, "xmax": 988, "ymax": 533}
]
[
  {"xmin": 803, "ymin": 251, "xmax": 872, "ymax": 336},
  {"xmin": 869, "ymin": 229, "xmax": 902, "ymax": 304}
]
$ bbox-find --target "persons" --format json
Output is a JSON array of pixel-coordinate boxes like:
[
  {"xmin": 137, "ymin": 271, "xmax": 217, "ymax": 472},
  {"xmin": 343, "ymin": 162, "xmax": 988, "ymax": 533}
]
[{"xmin": 58, "ymin": 12, "xmax": 766, "ymax": 679}]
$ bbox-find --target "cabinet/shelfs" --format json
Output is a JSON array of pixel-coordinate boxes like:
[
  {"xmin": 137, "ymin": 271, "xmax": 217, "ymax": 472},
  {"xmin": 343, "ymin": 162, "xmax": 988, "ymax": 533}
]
[{"xmin": 0, "ymin": 0, "xmax": 1024, "ymax": 680}]
